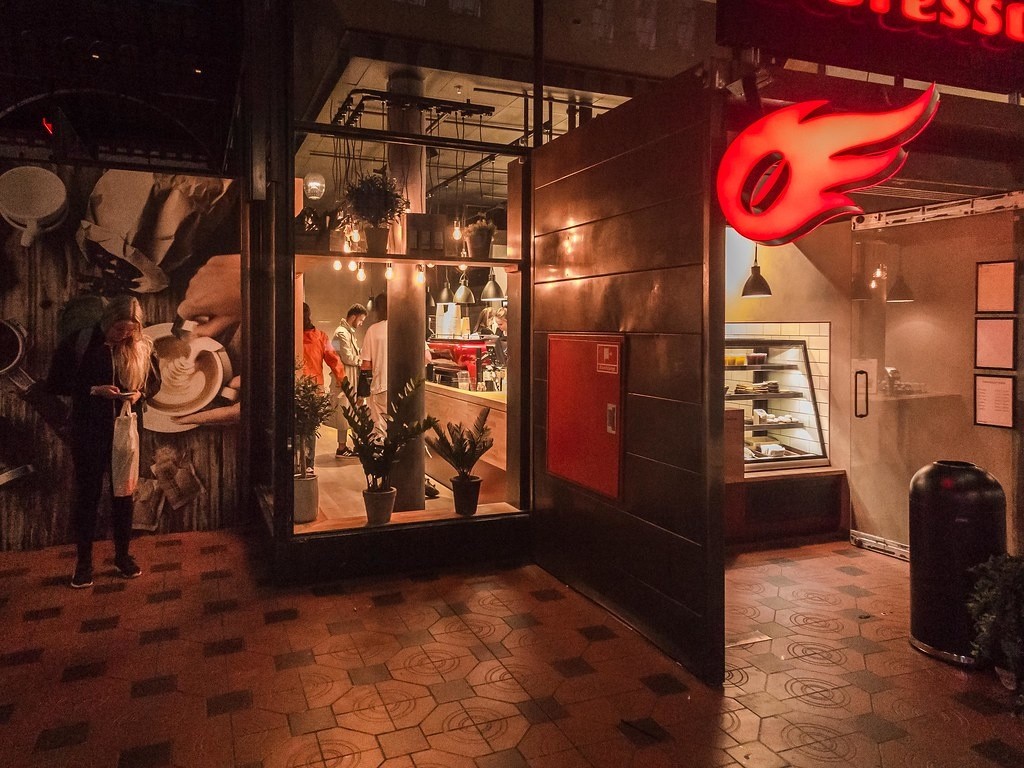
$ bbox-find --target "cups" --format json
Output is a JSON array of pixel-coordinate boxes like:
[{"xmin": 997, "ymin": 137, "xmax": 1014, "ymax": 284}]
[
  {"xmin": 0, "ymin": 166, "xmax": 68, "ymax": 235},
  {"xmin": 435, "ymin": 303, "xmax": 470, "ymax": 338},
  {"xmin": 724, "ymin": 352, "xmax": 767, "ymax": 366},
  {"xmin": 0, "ymin": 207, "xmax": 71, "ymax": 247},
  {"xmin": 458, "ymin": 370, "xmax": 470, "ymax": 391}
]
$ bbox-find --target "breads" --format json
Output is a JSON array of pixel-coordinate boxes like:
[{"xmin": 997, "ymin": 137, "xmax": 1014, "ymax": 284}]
[{"xmin": 765, "ymin": 413, "xmax": 800, "ymax": 424}]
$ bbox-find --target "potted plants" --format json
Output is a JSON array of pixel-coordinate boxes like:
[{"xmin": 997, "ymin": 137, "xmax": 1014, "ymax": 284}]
[
  {"xmin": 426, "ymin": 406, "xmax": 494, "ymax": 516},
  {"xmin": 293, "ymin": 352, "xmax": 338, "ymax": 523},
  {"xmin": 463, "ymin": 220, "xmax": 499, "ymax": 261},
  {"xmin": 965, "ymin": 554, "xmax": 1024, "ymax": 691},
  {"xmin": 339, "ymin": 374, "xmax": 440, "ymax": 524},
  {"xmin": 346, "ymin": 169, "xmax": 411, "ymax": 255}
]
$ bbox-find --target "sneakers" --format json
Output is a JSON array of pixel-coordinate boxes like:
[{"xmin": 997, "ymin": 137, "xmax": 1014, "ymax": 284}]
[
  {"xmin": 71, "ymin": 560, "xmax": 94, "ymax": 588},
  {"xmin": 113, "ymin": 555, "xmax": 142, "ymax": 578},
  {"xmin": 335, "ymin": 447, "xmax": 355, "ymax": 458}
]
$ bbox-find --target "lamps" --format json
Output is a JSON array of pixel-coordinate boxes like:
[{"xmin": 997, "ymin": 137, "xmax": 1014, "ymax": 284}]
[
  {"xmin": 741, "ymin": 242, "xmax": 772, "ymax": 299},
  {"xmin": 426, "ymin": 286, "xmax": 435, "ymax": 307},
  {"xmin": 454, "ymin": 176, "xmax": 476, "ymax": 303},
  {"xmin": 885, "ymin": 244, "xmax": 915, "ymax": 302},
  {"xmin": 438, "ymin": 184, "xmax": 456, "ymax": 303},
  {"xmin": 481, "ymin": 159, "xmax": 505, "ymax": 302}
]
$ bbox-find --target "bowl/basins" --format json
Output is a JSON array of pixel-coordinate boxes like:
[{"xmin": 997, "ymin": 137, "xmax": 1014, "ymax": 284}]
[
  {"xmin": 138, "ymin": 332, "xmax": 238, "ymax": 417},
  {"xmin": 0, "ymin": 319, "xmax": 24, "ymax": 375}
]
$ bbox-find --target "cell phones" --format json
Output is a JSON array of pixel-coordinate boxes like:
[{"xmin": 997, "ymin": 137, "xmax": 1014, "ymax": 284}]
[{"xmin": 119, "ymin": 391, "xmax": 136, "ymax": 395}]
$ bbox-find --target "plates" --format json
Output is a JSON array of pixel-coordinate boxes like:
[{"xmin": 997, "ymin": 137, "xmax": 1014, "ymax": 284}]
[{"xmin": 122, "ymin": 323, "xmax": 234, "ymax": 433}]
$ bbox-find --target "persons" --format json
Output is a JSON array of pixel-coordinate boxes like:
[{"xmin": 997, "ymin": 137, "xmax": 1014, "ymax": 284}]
[
  {"xmin": 360, "ymin": 292, "xmax": 439, "ymax": 497},
  {"xmin": 323, "ymin": 303, "xmax": 368, "ymax": 458},
  {"xmin": 473, "ymin": 306, "xmax": 507, "ymax": 357},
  {"xmin": 166, "ymin": 250, "xmax": 241, "ymax": 427},
  {"xmin": 45, "ymin": 296, "xmax": 161, "ymax": 588},
  {"xmin": 302, "ymin": 302, "xmax": 347, "ymax": 475}
]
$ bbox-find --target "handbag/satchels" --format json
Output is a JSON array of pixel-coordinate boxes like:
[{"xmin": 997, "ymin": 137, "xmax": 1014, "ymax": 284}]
[
  {"xmin": 112, "ymin": 400, "xmax": 139, "ymax": 498},
  {"xmin": 358, "ymin": 369, "xmax": 374, "ymax": 396}
]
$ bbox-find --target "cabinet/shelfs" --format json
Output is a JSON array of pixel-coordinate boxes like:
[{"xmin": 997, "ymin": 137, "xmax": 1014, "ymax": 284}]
[{"xmin": 722, "ymin": 338, "xmax": 829, "ymax": 472}]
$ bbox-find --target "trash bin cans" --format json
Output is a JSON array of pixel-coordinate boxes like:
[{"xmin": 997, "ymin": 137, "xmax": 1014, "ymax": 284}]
[{"xmin": 908, "ymin": 459, "xmax": 1007, "ymax": 663}]
